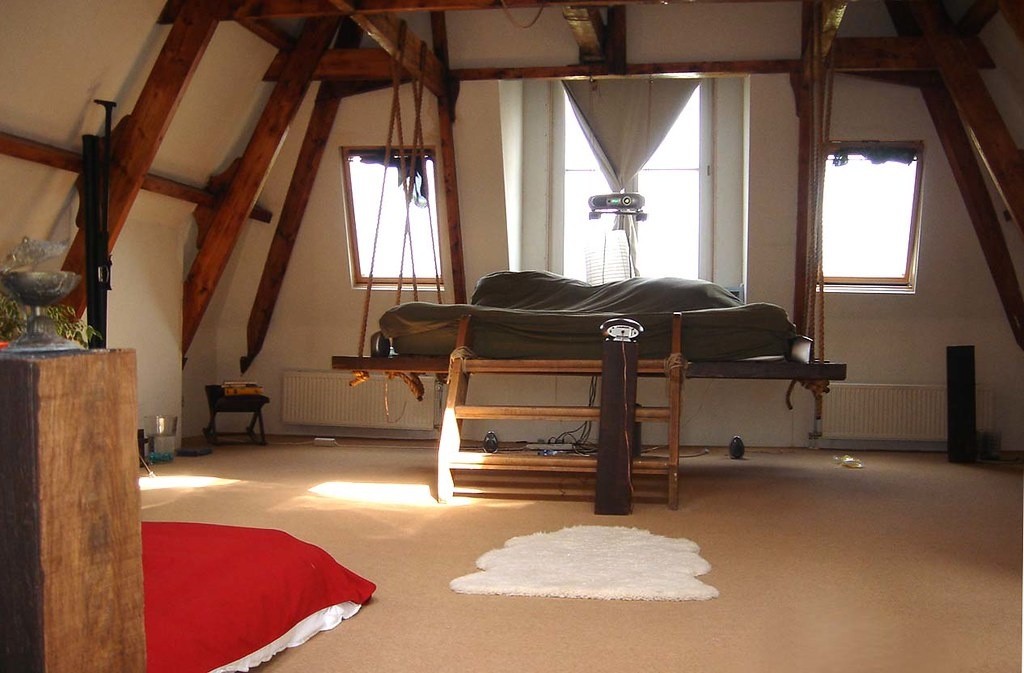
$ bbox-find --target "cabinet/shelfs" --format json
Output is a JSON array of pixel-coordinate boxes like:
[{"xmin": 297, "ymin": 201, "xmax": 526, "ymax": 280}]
[{"xmin": 1, "ymin": 349, "xmax": 145, "ymax": 672}]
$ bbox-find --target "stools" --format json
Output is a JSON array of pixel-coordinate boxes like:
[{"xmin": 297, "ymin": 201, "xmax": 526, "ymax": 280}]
[{"xmin": 201, "ymin": 383, "xmax": 270, "ymax": 443}]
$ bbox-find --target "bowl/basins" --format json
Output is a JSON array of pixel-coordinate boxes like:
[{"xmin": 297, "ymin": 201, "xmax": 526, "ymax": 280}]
[{"xmin": 144, "ymin": 415, "xmax": 177, "ymax": 436}]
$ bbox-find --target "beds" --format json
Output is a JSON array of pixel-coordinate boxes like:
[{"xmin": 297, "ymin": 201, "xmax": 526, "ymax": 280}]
[{"xmin": 142, "ymin": 520, "xmax": 376, "ymax": 673}]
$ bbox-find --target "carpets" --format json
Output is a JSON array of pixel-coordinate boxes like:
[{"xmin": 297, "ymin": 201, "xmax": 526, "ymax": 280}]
[{"xmin": 448, "ymin": 525, "xmax": 718, "ymax": 602}]
